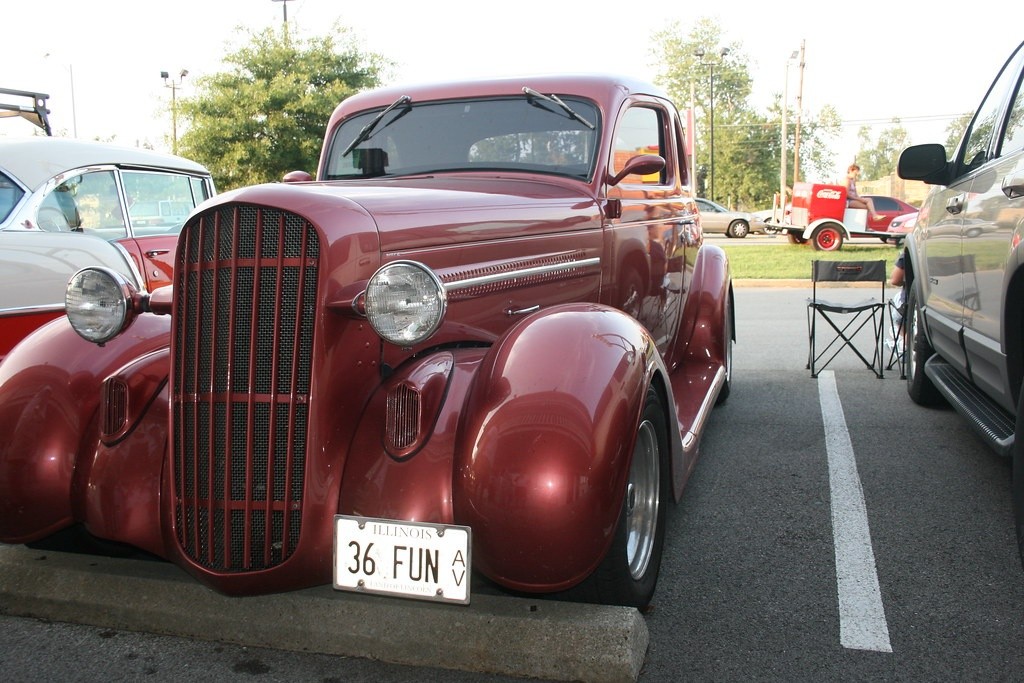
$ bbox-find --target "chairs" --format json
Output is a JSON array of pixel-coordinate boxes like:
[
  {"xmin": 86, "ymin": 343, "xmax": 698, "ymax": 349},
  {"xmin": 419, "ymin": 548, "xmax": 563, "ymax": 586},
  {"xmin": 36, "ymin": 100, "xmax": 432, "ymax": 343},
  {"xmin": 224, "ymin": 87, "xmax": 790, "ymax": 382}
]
[
  {"xmin": 805, "ymin": 258, "xmax": 888, "ymax": 379},
  {"xmin": 927, "ymin": 253, "xmax": 983, "ymax": 312},
  {"xmin": 884, "ymin": 247, "xmax": 915, "ymax": 380}
]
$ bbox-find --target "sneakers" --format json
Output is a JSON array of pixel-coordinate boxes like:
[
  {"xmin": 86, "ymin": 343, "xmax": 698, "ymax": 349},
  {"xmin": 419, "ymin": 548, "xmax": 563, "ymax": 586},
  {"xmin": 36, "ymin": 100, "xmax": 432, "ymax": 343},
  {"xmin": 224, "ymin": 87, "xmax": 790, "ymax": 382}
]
[
  {"xmin": 866, "ymin": 225, "xmax": 874, "ymax": 231},
  {"xmin": 884, "ymin": 337, "xmax": 908, "ymax": 363},
  {"xmin": 873, "ymin": 214, "xmax": 886, "ymax": 221},
  {"xmin": 889, "ymin": 326, "xmax": 905, "ymax": 341}
]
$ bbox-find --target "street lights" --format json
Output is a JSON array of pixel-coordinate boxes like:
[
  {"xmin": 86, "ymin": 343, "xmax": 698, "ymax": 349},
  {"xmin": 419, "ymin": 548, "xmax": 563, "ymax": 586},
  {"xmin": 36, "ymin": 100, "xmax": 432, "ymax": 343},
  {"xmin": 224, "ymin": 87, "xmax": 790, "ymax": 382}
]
[{"xmin": 161, "ymin": 68, "xmax": 189, "ymax": 156}]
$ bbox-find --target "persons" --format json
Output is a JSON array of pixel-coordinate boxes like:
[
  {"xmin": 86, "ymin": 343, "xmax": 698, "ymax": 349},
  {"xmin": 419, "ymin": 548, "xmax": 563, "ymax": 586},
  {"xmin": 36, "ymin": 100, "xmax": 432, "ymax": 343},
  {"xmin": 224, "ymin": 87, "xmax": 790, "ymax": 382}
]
[
  {"xmin": 891, "ymin": 251, "xmax": 918, "ymax": 362},
  {"xmin": 841, "ymin": 164, "xmax": 887, "ymax": 234}
]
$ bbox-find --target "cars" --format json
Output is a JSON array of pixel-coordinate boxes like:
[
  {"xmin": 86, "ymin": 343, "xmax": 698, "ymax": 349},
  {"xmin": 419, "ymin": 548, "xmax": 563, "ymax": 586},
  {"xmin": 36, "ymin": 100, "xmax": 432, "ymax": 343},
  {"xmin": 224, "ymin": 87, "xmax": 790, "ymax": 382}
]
[
  {"xmin": 0, "ymin": 72, "xmax": 739, "ymax": 611},
  {"xmin": 886, "ymin": 211, "xmax": 919, "ymax": 248},
  {"xmin": 921, "ymin": 217, "xmax": 999, "ymax": 240},
  {"xmin": 897, "ymin": 37, "xmax": 1024, "ymax": 566},
  {"xmin": 859, "ymin": 194, "xmax": 919, "ymax": 244},
  {"xmin": 694, "ymin": 199, "xmax": 765, "ymax": 238},
  {"xmin": 0, "ymin": 139, "xmax": 219, "ymax": 365},
  {"xmin": 753, "ymin": 202, "xmax": 791, "ymax": 236}
]
